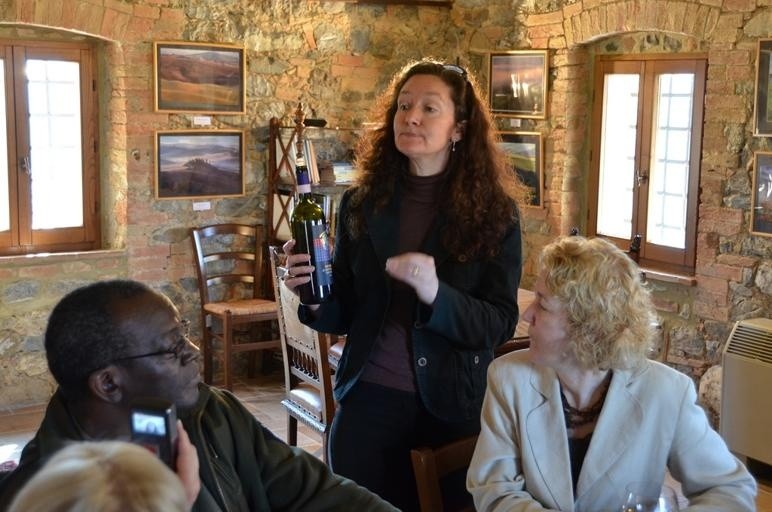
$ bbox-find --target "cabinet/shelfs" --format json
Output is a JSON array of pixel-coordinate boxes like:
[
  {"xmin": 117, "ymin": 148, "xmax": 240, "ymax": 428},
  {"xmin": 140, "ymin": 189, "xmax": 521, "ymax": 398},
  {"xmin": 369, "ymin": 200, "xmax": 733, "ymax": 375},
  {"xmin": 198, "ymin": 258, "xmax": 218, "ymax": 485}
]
[{"xmin": 268, "ymin": 116, "xmax": 363, "ymax": 244}]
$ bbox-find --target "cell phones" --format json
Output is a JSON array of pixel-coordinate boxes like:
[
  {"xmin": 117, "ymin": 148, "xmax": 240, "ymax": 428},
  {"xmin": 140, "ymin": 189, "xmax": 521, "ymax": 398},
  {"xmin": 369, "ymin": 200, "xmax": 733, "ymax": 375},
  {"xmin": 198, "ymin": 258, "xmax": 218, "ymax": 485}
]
[{"xmin": 126, "ymin": 397, "xmax": 178, "ymax": 474}]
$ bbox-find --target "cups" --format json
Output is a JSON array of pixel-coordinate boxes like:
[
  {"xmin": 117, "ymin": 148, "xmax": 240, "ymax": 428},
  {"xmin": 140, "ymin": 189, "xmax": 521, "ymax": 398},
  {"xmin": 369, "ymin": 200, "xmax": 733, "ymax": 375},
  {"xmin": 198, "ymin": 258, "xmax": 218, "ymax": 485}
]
[{"xmin": 619, "ymin": 480, "xmax": 678, "ymax": 512}]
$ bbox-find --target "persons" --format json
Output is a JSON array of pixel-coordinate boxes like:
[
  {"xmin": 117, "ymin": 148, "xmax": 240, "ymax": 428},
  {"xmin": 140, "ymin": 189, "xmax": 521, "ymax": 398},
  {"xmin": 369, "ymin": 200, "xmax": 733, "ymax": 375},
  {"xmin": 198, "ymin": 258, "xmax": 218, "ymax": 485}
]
[
  {"xmin": 464, "ymin": 236, "xmax": 757, "ymax": 512},
  {"xmin": 280, "ymin": 56, "xmax": 520, "ymax": 511},
  {"xmin": 1, "ymin": 281, "xmax": 405, "ymax": 512},
  {"xmin": 9, "ymin": 417, "xmax": 201, "ymax": 511},
  {"xmin": 144, "ymin": 421, "xmax": 160, "ymax": 433}
]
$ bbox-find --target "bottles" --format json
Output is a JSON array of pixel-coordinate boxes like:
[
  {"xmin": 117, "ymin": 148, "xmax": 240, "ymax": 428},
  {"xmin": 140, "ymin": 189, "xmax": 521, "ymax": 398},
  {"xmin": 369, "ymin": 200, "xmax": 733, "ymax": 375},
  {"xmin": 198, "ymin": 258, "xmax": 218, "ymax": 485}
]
[{"xmin": 289, "ymin": 153, "xmax": 335, "ymax": 307}]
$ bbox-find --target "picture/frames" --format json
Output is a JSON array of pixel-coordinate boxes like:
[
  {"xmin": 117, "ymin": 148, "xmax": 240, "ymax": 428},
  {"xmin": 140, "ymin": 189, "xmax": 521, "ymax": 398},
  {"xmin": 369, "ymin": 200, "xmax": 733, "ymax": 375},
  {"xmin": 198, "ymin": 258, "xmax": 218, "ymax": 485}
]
[
  {"xmin": 150, "ymin": 40, "xmax": 248, "ymax": 116},
  {"xmin": 152, "ymin": 127, "xmax": 248, "ymax": 201},
  {"xmin": 748, "ymin": 150, "xmax": 772, "ymax": 238},
  {"xmin": 486, "ymin": 49, "xmax": 549, "ymax": 119},
  {"xmin": 753, "ymin": 36, "xmax": 772, "ymax": 138},
  {"xmin": 495, "ymin": 130, "xmax": 545, "ymax": 211}
]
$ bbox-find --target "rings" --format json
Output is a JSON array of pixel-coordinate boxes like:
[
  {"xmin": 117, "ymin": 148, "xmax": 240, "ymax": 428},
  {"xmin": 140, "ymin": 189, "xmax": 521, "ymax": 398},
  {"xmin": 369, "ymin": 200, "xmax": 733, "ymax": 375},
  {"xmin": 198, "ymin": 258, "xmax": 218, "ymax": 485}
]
[
  {"xmin": 409, "ymin": 263, "xmax": 420, "ymax": 276},
  {"xmin": 284, "ymin": 268, "xmax": 296, "ymax": 279}
]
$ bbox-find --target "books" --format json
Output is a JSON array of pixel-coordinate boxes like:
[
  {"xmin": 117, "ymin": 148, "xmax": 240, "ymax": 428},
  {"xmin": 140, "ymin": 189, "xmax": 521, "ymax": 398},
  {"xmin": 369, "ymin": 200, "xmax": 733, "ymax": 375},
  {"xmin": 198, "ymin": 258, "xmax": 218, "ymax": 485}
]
[{"xmin": 293, "ymin": 139, "xmax": 355, "ymax": 185}]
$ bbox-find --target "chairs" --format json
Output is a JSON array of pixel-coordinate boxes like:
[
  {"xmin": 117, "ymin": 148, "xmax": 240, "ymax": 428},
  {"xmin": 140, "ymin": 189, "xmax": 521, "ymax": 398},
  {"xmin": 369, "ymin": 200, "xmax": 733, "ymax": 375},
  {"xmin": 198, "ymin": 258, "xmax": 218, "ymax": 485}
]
[
  {"xmin": 268, "ymin": 246, "xmax": 335, "ymax": 465},
  {"xmin": 410, "ymin": 432, "xmax": 480, "ymax": 512},
  {"xmin": 188, "ymin": 223, "xmax": 282, "ymax": 392}
]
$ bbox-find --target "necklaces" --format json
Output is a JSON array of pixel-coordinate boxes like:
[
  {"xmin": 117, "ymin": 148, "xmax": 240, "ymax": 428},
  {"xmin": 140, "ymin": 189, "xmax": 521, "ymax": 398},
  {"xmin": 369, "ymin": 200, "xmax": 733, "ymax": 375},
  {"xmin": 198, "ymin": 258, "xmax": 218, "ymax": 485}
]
[{"xmin": 561, "ymin": 371, "xmax": 613, "ymax": 428}]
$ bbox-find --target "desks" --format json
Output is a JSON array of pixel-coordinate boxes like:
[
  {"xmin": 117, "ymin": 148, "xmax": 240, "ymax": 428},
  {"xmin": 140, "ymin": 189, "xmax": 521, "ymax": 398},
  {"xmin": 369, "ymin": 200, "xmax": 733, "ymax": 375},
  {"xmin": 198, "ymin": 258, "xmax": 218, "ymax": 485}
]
[{"xmin": 495, "ymin": 288, "xmax": 536, "ymax": 354}]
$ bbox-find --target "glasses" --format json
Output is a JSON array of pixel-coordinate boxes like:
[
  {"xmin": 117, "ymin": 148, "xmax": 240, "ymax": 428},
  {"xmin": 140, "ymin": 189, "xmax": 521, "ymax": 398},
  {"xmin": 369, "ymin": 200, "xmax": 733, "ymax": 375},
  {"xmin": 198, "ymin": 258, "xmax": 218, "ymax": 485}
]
[
  {"xmin": 410, "ymin": 60, "xmax": 467, "ymax": 89},
  {"xmin": 85, "ymin": 318, "xmax": 191, "ymax": 375}
]
